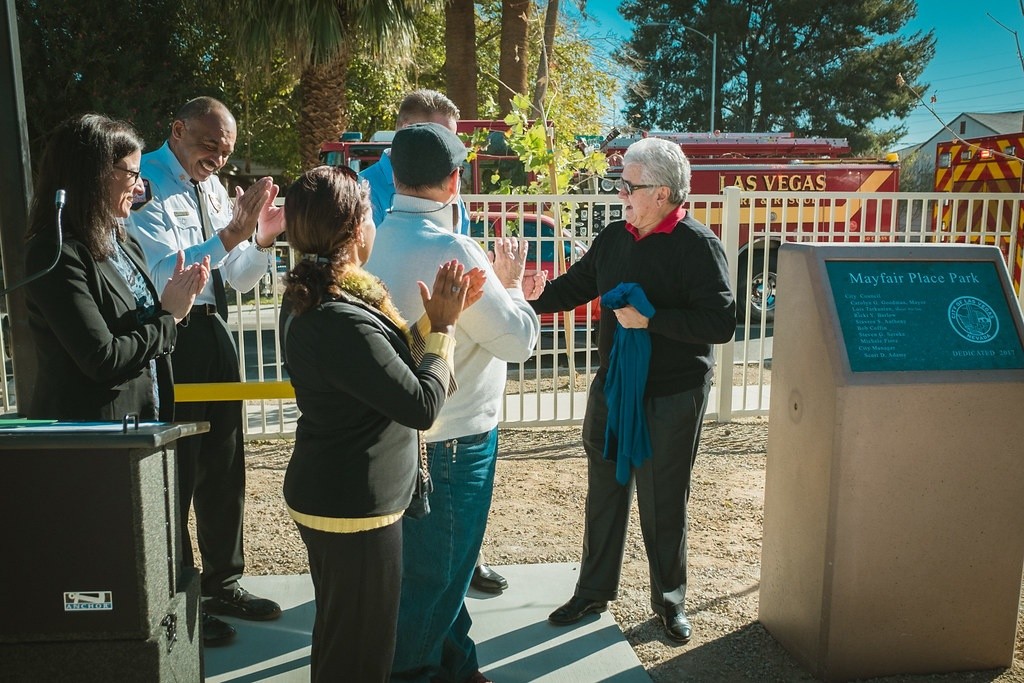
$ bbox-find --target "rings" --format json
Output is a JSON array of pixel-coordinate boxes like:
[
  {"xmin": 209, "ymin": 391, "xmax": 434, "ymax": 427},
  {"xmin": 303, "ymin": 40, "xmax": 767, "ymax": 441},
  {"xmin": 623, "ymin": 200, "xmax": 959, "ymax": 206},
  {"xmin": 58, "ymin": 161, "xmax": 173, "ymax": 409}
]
[{"xmin": 452, "ymin": 286, "xmax": 461, "ymax": 293}]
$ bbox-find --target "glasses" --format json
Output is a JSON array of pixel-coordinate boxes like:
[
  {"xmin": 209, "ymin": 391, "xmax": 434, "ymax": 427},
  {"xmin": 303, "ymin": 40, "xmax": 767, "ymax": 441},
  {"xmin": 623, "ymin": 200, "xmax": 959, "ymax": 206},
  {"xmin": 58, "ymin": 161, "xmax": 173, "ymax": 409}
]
[
  {"xmin": 619, "ymin": 178, "xmax": 666, "ymax": 195},
  {"xmin": 110, "ymin": 164, "xmax": 142, "ymax": 184},
  {"xmin": 445, "ymin": 165, "xmax": 466, "ymax": 178}
]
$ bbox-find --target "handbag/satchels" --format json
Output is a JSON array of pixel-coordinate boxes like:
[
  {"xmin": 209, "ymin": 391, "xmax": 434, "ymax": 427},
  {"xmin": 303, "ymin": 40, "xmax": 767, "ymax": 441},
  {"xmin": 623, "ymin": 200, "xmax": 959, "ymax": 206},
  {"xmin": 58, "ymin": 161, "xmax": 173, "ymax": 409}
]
[{"xmin": 403, "ymin": 468, "xmax": 433, "ymax": 520}]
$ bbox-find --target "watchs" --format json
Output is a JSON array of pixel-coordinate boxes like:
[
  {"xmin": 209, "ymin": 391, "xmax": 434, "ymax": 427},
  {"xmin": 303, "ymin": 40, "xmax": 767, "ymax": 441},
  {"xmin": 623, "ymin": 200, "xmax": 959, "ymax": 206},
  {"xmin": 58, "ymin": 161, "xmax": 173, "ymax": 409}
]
[{"xmin": 252, "ymin": 233, "xmax": 277, "ymax": 253}]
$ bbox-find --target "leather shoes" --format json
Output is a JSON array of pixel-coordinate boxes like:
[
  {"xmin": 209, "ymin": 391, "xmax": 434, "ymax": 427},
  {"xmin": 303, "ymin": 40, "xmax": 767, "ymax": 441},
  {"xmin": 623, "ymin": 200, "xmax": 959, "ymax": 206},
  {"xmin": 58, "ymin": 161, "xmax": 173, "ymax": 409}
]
[
  {"xmin": 469, "ymin": 562, "xmax": 507, "ymax": 593},
  {"xmin": 202, "ymin": 589, "xmax": 281, "ymax": 621},
  {"xmin": 658, "ymin": 611, "xmax": 691, "ymax": 641},
  {"xmin": 548, "ymin": 597, "xmax": 606, "ymax": 625},
  {"xmin": 201, "ymin": 613, "xmax": 236, "ymax": 645}
]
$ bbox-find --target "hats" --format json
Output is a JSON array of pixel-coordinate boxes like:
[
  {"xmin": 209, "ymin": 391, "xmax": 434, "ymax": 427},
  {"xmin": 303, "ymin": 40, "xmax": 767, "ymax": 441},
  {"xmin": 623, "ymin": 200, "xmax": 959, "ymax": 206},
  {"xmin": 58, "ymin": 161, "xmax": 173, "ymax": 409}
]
[{"xmin": 389, "ymin": 123, "xmax": 468, "ymax": 184}]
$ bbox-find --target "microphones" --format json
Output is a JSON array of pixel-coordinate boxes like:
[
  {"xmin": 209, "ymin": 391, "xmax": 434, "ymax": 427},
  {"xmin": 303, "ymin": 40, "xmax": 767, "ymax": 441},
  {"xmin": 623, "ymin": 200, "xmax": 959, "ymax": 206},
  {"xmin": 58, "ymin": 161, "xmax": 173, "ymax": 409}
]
[{"xmin": 0, "ymin": 190, "xmax": 67, "ymax": 298}]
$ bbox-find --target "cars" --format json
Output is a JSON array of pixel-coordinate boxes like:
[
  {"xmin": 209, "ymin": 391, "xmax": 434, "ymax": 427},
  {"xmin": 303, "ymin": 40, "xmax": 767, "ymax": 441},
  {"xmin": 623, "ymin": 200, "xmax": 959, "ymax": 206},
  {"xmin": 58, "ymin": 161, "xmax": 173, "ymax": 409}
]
[{"xmin": 466, "ymin": 210, "xmax": 601, "ymax": 345}]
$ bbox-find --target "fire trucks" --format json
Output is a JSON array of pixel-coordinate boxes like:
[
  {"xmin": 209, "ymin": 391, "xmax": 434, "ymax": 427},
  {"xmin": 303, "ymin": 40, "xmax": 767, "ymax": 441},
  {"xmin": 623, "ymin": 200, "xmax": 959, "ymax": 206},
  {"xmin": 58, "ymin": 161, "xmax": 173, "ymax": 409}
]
[
  {"xmin": 933, "ymin": 132, "xmax": 1024, "ymax": 300},
  {"xmin": 319, "ymin": 117, "xmax": 902, "ymax": 325}
]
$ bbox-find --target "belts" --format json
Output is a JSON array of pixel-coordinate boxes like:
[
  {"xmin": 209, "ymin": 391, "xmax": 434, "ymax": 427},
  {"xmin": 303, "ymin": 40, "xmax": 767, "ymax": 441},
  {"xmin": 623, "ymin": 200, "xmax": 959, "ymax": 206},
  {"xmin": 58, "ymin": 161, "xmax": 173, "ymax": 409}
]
[{"xmin": 189, "ymin": 304, "xmax": 219, "ymax": 315}]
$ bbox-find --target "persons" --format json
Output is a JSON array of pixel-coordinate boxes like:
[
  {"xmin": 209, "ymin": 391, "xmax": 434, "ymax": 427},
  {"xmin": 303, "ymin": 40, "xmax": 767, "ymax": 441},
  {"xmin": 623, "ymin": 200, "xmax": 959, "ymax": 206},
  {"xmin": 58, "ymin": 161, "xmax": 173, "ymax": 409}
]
[
  {"xmin": 354, "ymin": 122, "xmax": 541, "ymax": 683},
  {"xmin": 275, "ymin": 168, "xmax": 488, "ymax": 683},
  {"xmin": 358, "ymin": 87, "xmax": 509, "ymax": 596},
  {"xmin": 118, "ymin": 96, "xmax": 287, "ymax": 643},
  {"xmin": 522, "ymin": 137, "xmax": 737, "ymax": 644},
  {"xmin": 9, "ymin": 108, "xmax": 210, "ymax": 427}
]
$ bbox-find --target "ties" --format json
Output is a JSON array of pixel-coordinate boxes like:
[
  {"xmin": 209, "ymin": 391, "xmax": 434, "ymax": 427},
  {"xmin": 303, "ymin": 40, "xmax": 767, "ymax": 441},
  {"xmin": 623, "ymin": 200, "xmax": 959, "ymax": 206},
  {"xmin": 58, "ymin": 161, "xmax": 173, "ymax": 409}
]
[{"xmin": 191, "ymin": 180, "xmax": 228, "ymax": 322}]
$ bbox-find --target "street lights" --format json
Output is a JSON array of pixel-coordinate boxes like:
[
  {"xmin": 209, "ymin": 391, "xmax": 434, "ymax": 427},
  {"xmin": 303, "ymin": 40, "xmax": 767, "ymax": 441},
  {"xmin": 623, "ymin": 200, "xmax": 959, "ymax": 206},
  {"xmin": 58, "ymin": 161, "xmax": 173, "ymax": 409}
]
[{"xmin": 641, "ymin": 22, "xmax": 716, "ymax": 134}]
